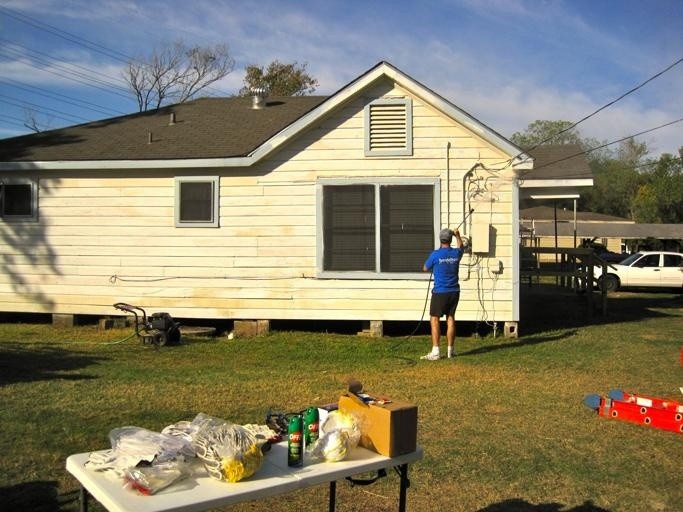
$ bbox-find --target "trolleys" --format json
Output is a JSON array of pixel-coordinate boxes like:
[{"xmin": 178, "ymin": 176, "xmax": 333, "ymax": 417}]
[{"xmin": 112, "ymin": 302, "xmax": 181, "ymax": 347}]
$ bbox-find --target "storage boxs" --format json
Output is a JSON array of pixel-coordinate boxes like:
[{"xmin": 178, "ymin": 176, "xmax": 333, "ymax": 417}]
[{"xmin": 337, "ymin": 381, "xmax": 417, "ymax": 458}]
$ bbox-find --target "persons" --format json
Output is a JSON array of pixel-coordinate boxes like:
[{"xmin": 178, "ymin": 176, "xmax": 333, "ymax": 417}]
[{"xmin": 418, "ymin": 227, "xmax": 464, "ymax": 361}]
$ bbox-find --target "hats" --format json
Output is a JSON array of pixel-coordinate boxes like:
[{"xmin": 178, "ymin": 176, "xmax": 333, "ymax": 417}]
[{"xmin": 440, "ymin": 228, "xmax": 452, "ymax": 243}]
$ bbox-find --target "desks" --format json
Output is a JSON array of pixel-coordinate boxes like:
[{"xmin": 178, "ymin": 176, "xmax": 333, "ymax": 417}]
[{"xmin": 64, "ymin": 431, "xmax": 423, "ymax": 512}]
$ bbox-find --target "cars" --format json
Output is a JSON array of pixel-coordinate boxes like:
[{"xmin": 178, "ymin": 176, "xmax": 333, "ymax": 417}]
[{"xmin": 571, "ymin": 251, "xmax": 683, "ymax": 292}]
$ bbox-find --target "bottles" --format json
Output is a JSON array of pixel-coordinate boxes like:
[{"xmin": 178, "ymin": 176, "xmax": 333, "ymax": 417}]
[
  {"xmin": 287, "ymin": 414, "xmax": 304, "ymax": 468},
  {"xmin": 303, "ymin": 406, "xmax": 319, "ymax": 449}
]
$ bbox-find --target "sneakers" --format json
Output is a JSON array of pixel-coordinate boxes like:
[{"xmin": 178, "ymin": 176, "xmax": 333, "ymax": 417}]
[
  {"xmin": 448, "ymin": 351, "xmax": 458, "ymax": 358},
  {"xmin": 419, "ymin": 353, "xmax": 439, "ymax": 361}
]
